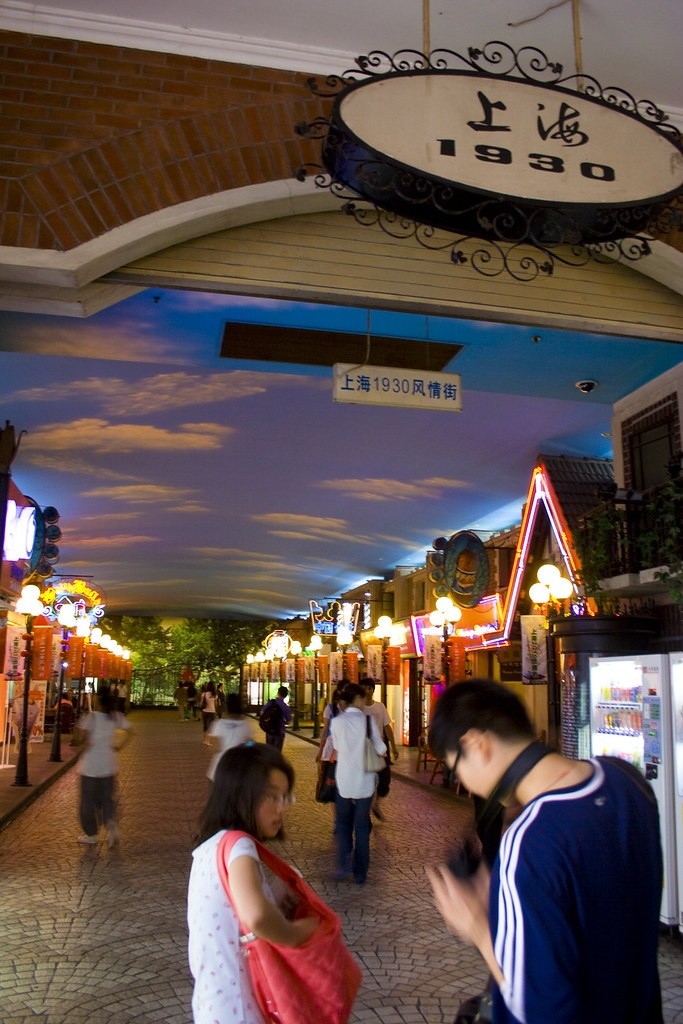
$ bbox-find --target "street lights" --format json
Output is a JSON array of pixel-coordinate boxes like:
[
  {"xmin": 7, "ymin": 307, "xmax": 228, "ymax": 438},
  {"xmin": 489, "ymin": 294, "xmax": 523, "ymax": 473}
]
[
  {"xmin": 47, "ymin": 602, "xmax": 78, "ymax": 763},
  {"xmin": 374, "ymin": 616, "xmax": 394, "ymax": 708},
  {"xmin": 308, "ymin": 636, "xmax": 323, "ymax": 740},
  {"xmin": 11, "ymin": 584, "xmax": 44, "ymax": 787},
  {"xmin": 429, "ymin": 596, "xmax": 462, "ymax": 690},
  {"xmin": 337, "ymin": 626, "xmax": 354, "ymax": 682},
  {"xmin": 246, "ymin": 644, "xmax": 287, "ymax": 718},
  {"xmin": 290, "ymin": 640, "xmax": 303, "ymax": 731},
  {"xmin": 528, "ymin": 563, "xmax": 573, "ymax": 754},
  {"xmin": 70, "ymin": 617, "xmax": 131, "ymax": 744}
]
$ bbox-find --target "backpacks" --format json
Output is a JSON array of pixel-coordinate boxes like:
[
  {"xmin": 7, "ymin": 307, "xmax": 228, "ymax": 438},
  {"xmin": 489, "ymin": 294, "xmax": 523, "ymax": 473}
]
[{"xmin": 259, "ymin": 699, "xmax": 287, "ymax": 737}]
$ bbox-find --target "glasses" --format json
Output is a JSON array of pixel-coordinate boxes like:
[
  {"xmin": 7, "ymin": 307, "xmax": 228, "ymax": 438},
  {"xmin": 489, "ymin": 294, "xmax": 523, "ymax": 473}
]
[
  {"xmin": 441, "ymin": 744, "xmax": 463, "ymax": 793},
  {"xmin": 259, "ymin": 786, "xmax": 297, "ymax": 808}
]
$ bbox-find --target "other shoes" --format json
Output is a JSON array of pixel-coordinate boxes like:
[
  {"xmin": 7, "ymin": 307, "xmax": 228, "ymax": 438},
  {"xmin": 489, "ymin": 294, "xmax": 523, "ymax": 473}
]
[
  {"xmin": 77, "ymin": 834, "xmax": 98, "ymax": 844},
  {"xmin": 317, "ymin": 868, "xmax": 350, "ymax": 882},
  {"xmin": 203, "ymin": 741, "xmax": 213, "ymax": 747},
  {"xmin": 371, "ymin": 804, "xmax": 386, "ymax": 822},
  {"xmin": 108, "ymin": 828, "xmax": 122, "ymax": 849},
  {"xmin": 353, "ymin": 873, "xmax": 369, "ymax": 884}
]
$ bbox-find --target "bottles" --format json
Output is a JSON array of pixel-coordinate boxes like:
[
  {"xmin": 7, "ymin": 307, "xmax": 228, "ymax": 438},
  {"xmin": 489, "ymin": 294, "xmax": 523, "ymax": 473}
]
[{"xmin": 598, "ymin": 685, "xmax": 644, "ymax": 776}]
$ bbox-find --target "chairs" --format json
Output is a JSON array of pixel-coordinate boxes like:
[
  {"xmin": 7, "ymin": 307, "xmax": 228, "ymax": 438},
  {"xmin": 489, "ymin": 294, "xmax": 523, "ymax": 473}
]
[
  {"xmin": 55, "ymin": 703, "xmax": 75, "ymax": 735},
  {"xmin": 416, "ymin": 725, "xmax": 472, "ymax": 799}
]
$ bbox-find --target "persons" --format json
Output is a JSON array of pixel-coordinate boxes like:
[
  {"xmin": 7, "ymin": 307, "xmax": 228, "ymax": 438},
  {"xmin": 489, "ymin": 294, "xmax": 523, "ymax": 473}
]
[
  {"xmin": 187, "ymin": 741, "xmax": 330, "ymax": 1024},
  {"xmin": 472, "ymin": 793, "xmax": 503, "ymax": 857},
  {"xmin": 428, "ymin": 679, "xmax": 665, "ymax": 1024},
  {"xmin": 329, "ymin": 684, "xmax": 388, "ymax": 884},
  {"xmin": 53, "ymin": 678, "xmax": 399, "ymax": 845}
]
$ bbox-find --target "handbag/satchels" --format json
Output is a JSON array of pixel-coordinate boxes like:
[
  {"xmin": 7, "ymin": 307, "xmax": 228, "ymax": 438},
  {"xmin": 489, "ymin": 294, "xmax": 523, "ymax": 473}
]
[
  {"xmin": 200, "ymin": 691, "xmax": 208, "ymax": 709},
  {"xmin": 111, "ymin": 710, "xmax": 130, "ymax": 748},
  {"xmin": 364, "ymin": 715, "xmax": 387, "ymax": 772},
  {"xmin": 217, "ymin": 827, "xmax": 363, "ymax": 1024},
  {"xmin": 453, "ymin": 984, "xmax": 494, "ymax": 1024},
  {"xmin": 315, "ymin": 748, "xmax": 338, "ymax": 803}
]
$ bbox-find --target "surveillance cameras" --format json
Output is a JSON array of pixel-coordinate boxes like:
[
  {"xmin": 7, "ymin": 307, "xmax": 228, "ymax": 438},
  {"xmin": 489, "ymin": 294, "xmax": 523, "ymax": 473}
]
[{"xmin": 575, "ymin": 380, "xmax": 599, "ymax": 394}]
